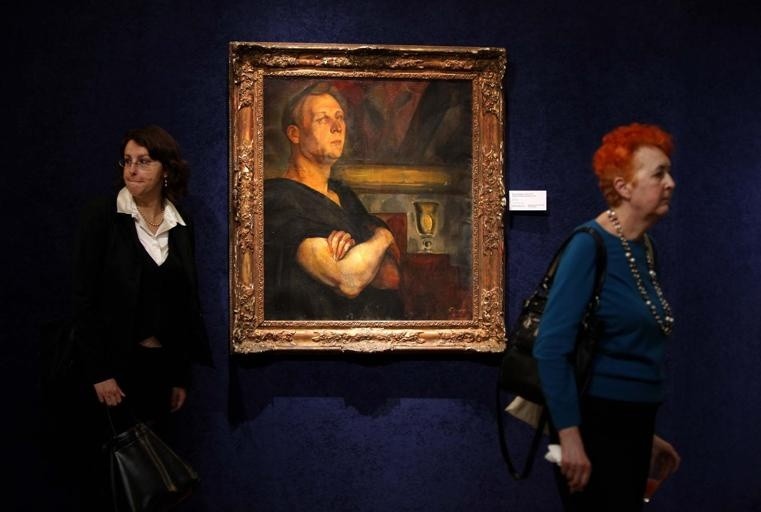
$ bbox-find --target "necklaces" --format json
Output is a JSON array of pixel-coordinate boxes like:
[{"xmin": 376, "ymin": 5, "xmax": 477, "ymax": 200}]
[
  {"xmin": 135, "ymin": 200, "xmax": 165, "ymax": 227},
  {"xmin": 605, "ymin": 207, "xmax": 675, "ymax": 339}
]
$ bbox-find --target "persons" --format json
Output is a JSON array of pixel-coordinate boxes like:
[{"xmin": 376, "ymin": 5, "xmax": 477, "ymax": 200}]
[
  {"xmin": 532, "ymin": 120, "xmax": 684, "ymax": 510},
  {"xmin": 86, "ymin": 124, "xmax": 218, "ymax": 512},
  {"xmin": 264, "ymin": 84, "xmax": 406, "ymax": 319}
]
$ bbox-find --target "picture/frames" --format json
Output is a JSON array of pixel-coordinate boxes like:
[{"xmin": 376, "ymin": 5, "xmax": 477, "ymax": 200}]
[{"xmin": 226, "ymin": 40, "xmax": 511, "ymax": 359}]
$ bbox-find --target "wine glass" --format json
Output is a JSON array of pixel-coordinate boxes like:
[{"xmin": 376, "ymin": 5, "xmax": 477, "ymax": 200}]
[{"xmin": 414, "ymin": 201, "xmax": 439, "ymax": 250}]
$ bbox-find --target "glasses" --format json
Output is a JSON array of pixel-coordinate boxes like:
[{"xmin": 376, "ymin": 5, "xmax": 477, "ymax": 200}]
[{"xmin": 118, "ymin": 157, "xmax": 155, "ymax": 171}]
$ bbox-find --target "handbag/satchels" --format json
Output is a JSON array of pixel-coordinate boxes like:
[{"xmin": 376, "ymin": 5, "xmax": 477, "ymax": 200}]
[
  {"xmin": 84, "ymin": 395, "xmax": 205, "ymax": 511},
  {"xmin": 494, "ymin": 225, "xmax": 607, "ymax": 480}
]
